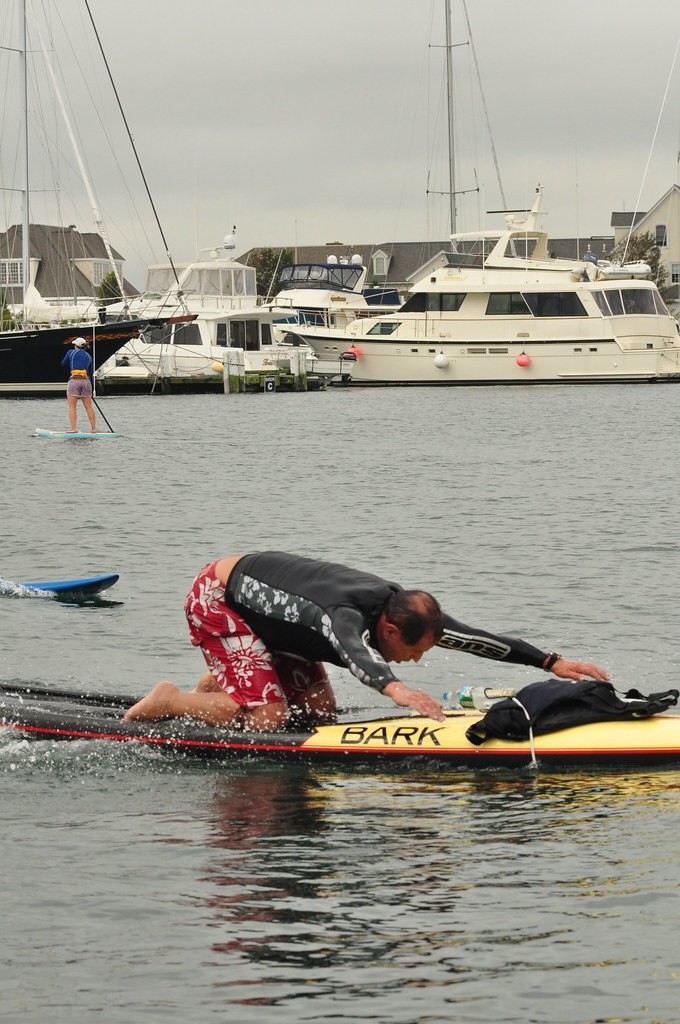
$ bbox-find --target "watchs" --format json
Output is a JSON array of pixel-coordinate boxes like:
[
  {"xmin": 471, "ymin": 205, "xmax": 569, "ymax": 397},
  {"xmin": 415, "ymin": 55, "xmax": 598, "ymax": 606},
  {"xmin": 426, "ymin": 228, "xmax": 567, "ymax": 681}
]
[{"xmin": 544, "ymin": 649, "xmax": 562, "ymax": 672}]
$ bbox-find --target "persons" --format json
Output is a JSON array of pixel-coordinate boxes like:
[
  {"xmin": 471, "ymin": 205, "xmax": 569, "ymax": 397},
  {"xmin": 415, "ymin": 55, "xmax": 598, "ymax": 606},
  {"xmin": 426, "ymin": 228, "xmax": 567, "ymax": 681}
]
[
  {"xmin": 61, "ymin": 337, "xmax": 97, "ymax": 432},
  {"xmin": 122, "ymin": 549, "xmax": 612, "ymax": 733}
]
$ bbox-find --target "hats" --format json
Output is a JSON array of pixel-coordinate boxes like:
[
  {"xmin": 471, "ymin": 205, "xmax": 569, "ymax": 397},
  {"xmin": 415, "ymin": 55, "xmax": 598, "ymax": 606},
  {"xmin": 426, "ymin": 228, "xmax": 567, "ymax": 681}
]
[{"xmin": 72, "ymin": 338, "xmax": 87, "ymax": 347}]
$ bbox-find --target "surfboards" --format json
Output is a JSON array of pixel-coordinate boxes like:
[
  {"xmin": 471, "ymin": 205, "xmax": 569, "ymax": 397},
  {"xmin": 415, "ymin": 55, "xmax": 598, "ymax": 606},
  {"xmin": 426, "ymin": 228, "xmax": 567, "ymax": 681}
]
[
  {"xmin": 1, "ymin": 683, "xmax": 680, "ymax": 757},
  {"xmin": 0, "ymin": 574, "xmax": 120, "ymax": 593},
  {"xmin": 34, "ymin": 427, "xmax": 120, "ymax": 438}
]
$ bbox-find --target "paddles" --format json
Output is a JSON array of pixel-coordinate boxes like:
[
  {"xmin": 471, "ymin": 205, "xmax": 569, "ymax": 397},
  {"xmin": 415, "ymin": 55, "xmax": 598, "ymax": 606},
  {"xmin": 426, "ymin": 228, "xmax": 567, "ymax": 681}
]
[{"xmin": 91, "ymin": 396, "xmax": 115, "ymax": 433}]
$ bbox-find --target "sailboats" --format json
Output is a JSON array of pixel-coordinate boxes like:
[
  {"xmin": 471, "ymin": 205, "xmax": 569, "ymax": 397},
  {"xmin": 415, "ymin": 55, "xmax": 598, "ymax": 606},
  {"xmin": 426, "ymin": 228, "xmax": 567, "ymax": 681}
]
[{"xmin": 0, "ymin": 0, "xmax": 200, "ymax": 399}]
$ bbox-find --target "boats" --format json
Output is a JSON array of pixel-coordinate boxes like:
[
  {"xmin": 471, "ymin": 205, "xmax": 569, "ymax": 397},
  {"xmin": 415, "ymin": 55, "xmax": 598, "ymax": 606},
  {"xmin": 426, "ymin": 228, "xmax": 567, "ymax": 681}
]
[
  {"xmin": 269, "ymin": 352, "xmax": 355, "ymax": 377},
  {"xmin": 263, "ymin": 255, "xmax": 405, "ymax": 348},
  {"xmin": 281, "ymin": 181, "xmax": 680, "ymax": 384},
  {"xmin": 571, "ymin": 258, "xmax": 652, "ymax": 282},
  {"xmin": 93, "ymin": 225, "xmax": 316, "ymax": 376}
]
